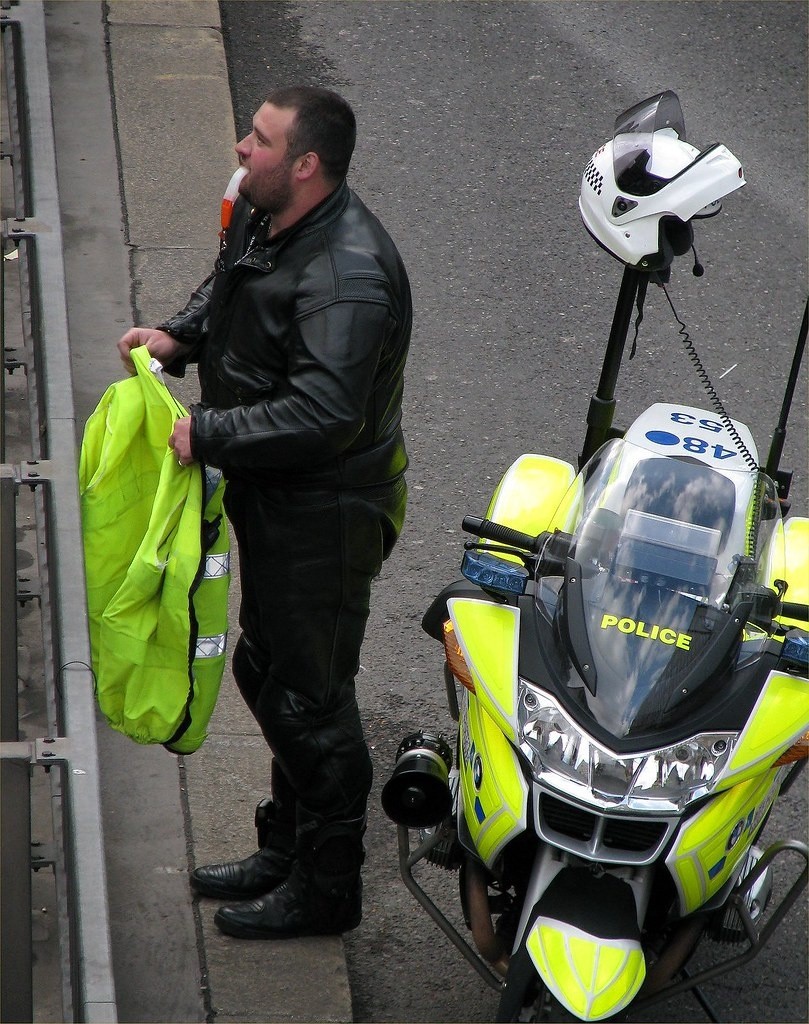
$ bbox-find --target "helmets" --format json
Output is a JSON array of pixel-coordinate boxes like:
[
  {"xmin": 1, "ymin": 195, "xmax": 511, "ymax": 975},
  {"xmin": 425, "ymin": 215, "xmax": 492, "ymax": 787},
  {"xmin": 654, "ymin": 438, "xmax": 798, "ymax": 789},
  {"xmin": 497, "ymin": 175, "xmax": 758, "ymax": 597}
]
[{"xmin": 579, "ymin": 90, "xmax": 747, "ymax": 274}]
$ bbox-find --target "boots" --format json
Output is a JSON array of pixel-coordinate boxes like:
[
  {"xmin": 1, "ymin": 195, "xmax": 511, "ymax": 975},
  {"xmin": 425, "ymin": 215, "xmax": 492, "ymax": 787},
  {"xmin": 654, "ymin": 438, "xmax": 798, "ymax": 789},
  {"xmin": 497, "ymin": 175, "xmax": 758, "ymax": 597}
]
[
  {"xmin": 215, "ymin": 803, "xmax": 367, "ymax": 940},
  {"xmin": 191, "ymin": 757, "xmax": 294, "ymax": 900}
]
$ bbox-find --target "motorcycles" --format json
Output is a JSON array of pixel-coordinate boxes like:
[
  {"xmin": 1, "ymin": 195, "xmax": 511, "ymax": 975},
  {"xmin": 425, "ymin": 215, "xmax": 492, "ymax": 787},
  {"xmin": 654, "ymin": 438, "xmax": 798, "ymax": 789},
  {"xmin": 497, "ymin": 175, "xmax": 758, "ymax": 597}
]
[{"xmin": 382, "ymin": 268, "xmax": 809, "ymax": 1024}]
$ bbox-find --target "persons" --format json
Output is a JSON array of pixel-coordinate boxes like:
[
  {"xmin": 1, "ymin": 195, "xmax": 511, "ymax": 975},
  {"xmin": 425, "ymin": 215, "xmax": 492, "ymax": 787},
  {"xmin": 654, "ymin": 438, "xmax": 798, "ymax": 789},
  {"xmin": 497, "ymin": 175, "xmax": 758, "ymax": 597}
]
[{"xmin": 116, "ymin": 86, "xmax": 413, "ymax": 940}]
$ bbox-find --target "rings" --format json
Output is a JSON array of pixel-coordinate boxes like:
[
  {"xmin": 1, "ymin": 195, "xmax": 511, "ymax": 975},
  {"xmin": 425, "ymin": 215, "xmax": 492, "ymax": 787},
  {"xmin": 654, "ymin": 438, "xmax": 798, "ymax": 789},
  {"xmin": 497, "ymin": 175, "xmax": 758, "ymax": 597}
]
[{"xmin": 178, "ymin": 461, "xmax": 186, "ymax": 467}]
